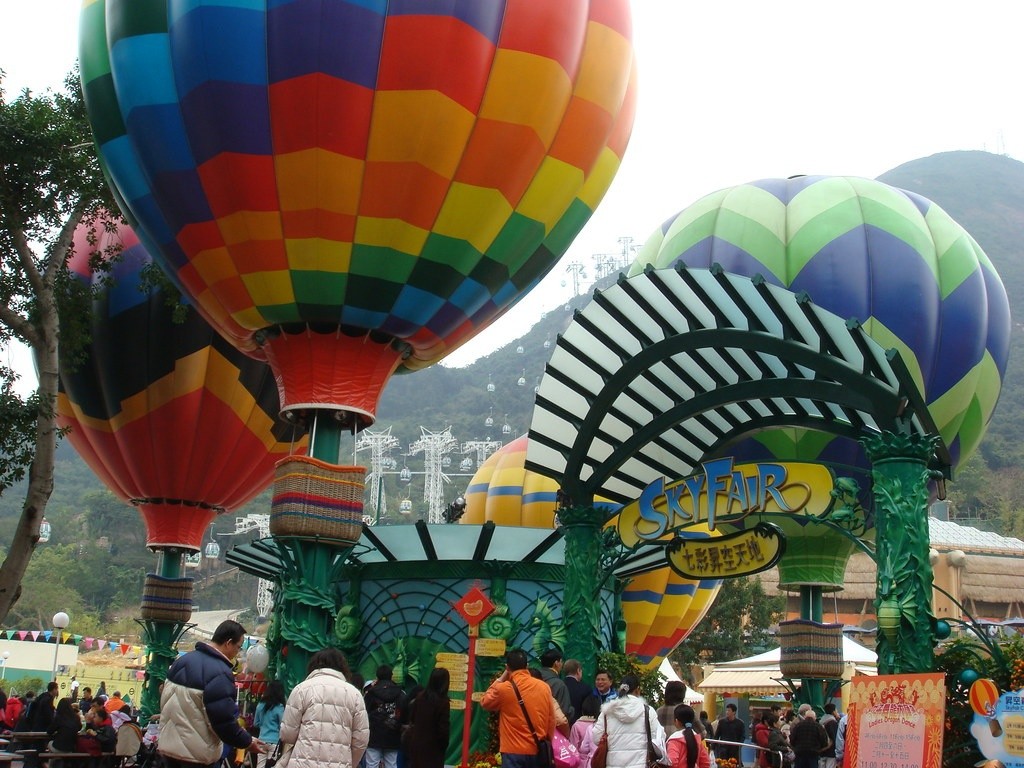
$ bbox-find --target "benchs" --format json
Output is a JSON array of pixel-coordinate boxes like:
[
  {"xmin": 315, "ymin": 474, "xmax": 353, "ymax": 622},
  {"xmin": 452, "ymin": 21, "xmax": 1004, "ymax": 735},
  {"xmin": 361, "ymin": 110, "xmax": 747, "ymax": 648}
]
[
  {"xmin": 37, "ymin": 751, "xmax": 127, "ymax": 758},
  {"xmin": 17, "ymin": 748, "xmax": 54, "ymax": 754}
]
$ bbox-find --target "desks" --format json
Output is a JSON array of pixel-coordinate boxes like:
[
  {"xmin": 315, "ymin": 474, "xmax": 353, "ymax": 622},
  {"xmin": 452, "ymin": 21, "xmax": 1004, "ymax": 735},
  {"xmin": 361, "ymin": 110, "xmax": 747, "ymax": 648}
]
[{"xmin": 13, "ymin": 732, "xmax": 87, "ymax": 748}]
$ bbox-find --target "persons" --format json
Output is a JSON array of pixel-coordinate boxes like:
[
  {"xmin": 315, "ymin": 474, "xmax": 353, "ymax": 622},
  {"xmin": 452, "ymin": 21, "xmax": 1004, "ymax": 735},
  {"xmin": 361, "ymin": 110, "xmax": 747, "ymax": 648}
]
[
  {"xmin": 527, "ymin": 649, "xmax": 618, "ymax": 768},
  {"xmin": 592, "ymin": 674, "xmax": 667, "ymax": 768},
  {"xmin": 750, "ymin": 701, "xmax": 847, "ymax": 768},
  {"xmin": 713, "ymin": 703, "xmax": 745, "ymax": 765},
  {"xmin": 279, "ymin": 648, "xmax": 370, "ymax": 768},
  {"xmin": 698, "ymin": 711, "xmax": 714, "ymax": 750},
  {"xmin": 253, "ymin": 679, "xmax": 286, "ymax": 768},
  {"xmin": 0, "ymin": 677, "xmax": 165, "ymax": 768},
  {"xmin": 405, "ymin": 668, "xmax": 450, "ymax": 768},
  {"xmin": 480, "ymin": 648, "xmax": 556, "ymax": 768},
  {"xmin": 156, "ymin": 620, "xmax": 267, "ymax": 768},
  {"xmin": 664, "ymin": 704, "xmax": 711, "ymax": 768},
  {"xmin": 351, "ymin": 665, "xmax": 424, "ymax": 768},
  {"xmin": 656, "ymin": 681, "xmax": 707, "ymax": 743}
]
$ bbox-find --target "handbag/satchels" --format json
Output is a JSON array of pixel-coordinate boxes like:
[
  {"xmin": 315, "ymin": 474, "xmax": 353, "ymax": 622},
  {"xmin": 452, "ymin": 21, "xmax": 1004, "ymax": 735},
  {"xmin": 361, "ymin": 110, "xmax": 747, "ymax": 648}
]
[
  {"xmin": 552, "ymin": 728, "xmax": 580, "ymax": 768},
  {"xmin": 590, "ymin": 732, "xmax": 608, "ymax": 768},
  {"xmin": 537, "ymin": 740, "xmax": 552, "ymax": 767},
  {"xmin": 25, "ymin": 701, "xmax": 36, "ymax": 719},
  {"xmin": 265, "ymin": 738, "xmax": 282, "ymax": 768},
  {"xmin": 646, "ymin": 742, "xmax": 665, "ymax": 768},
  {"xmin": 74, "ymin": 734, "xmax": 101, "ymax": 756},
  {"xmin": 783, "ymin": 750, "xmax": 796, "ymax": 766}
]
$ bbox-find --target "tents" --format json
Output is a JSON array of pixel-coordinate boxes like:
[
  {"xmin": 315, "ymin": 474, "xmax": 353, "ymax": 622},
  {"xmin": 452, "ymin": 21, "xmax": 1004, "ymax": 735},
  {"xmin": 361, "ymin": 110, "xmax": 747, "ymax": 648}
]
[
  {"xmin": 652, "ymin": 658, "xmax": 704, "ymax": 705},
  {"xmin": 712, "ymin": 623, "xmax": 882, "ymax": 679}
]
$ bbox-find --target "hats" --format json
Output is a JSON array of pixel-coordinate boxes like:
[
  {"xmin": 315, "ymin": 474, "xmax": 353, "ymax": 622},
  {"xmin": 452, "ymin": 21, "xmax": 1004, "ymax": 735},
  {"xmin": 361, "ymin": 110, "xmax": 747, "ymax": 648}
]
[{"xmin": 9, "ymin": 687, "xmax": 18, "ymax": 697}]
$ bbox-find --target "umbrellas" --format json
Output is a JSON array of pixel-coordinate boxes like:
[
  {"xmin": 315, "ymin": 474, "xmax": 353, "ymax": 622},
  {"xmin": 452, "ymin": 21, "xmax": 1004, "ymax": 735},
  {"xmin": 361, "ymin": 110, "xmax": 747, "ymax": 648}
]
[{"xmin": 841, "ymin": 616, "xmax": 1024, "ymax": 640}]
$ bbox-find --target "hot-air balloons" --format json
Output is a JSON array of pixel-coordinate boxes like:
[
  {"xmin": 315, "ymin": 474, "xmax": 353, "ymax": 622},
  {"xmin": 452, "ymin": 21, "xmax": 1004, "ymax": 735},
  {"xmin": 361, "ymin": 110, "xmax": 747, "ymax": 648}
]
[
  {"xmin": 31, "ymin": 201, "xmax": 311, "ymax": 623},
  {"xmin": 458, "ymin": 431, "xmax": 724, "ymax": 695},
  {"xmin": 77, "ymin": 1, "xmax": 640, "ymax": 548},
  {"xmin": 624, "ymin": 172, "xmax": 1012, "ymax": 679}
]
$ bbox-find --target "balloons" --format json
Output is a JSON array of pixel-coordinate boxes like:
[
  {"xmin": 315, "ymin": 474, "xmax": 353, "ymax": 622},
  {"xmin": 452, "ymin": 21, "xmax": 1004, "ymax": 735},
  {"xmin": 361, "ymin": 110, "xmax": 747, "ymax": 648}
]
[{"xmin": 230, "ymin": 645, "xmax": 267, "ymax": 694}]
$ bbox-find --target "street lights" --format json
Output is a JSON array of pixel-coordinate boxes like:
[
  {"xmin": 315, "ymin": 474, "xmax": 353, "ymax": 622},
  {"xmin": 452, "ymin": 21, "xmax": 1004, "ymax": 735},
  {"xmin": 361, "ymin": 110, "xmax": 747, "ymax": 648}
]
[
  {"xmin": 2, "ymin": 650, "xmax": 11, "ymax": 680},
  {"xmin": 52, "ymin": 612, "xmax": 70, "ymax": 684}
]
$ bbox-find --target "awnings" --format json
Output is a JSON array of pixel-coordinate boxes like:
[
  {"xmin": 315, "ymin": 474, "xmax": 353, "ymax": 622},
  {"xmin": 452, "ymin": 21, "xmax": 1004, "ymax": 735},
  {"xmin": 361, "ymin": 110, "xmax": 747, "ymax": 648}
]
[{"xmin": 698, "ymin": 670, "xmax": 801, "ymax": 695}]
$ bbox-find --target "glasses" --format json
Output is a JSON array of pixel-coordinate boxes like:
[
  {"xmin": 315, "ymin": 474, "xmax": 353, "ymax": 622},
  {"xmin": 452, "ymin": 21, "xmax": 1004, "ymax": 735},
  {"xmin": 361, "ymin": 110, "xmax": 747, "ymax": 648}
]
[{"xmin": 233, "ymin": 641, "xmax": 243, "ymax": 653}]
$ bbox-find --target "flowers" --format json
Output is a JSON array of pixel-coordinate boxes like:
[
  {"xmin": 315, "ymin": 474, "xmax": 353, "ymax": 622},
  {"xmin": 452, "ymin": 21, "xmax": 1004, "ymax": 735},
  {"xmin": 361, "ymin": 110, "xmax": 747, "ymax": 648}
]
[
  {"xmin": 454, "ymin": 750, "xmax": 502, "ymax": 768},
  {"xmin": 715, "ymin": 757, "xmax": 737, "ymax": 768}
]
